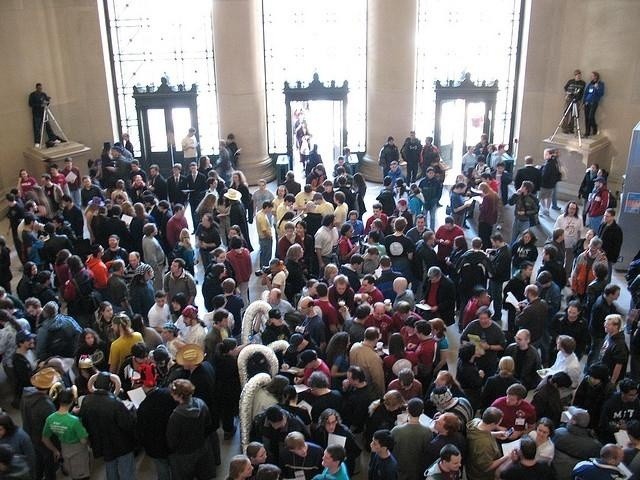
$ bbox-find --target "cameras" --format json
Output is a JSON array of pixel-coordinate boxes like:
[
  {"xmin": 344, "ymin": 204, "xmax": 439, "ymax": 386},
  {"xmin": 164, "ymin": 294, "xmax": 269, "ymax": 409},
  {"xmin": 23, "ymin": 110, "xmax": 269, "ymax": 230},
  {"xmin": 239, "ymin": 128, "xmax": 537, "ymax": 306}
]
[{"xmin": 255, "ymin": 266, "xmax": 271, "ymax": 276}]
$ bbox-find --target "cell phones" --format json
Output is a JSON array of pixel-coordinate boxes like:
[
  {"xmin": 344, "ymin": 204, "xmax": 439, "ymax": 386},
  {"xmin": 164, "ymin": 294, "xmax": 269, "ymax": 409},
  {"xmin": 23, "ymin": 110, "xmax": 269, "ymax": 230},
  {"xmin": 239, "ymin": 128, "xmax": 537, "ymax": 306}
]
[{"xmin": 506, "ymin": 427, "xmax": 514, "ymax": 437}]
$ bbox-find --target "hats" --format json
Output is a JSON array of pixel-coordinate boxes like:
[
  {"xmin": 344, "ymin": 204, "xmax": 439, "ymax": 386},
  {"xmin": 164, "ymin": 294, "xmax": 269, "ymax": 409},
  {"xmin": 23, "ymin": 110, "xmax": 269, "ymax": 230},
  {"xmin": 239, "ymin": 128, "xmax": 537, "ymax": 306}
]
[
  {"xmin": 428, "ymin": 385, "xmax": 455, "ymax": 409},
  {"xmin": 175, "ymin": 343, "xmax": 205, "ymax": 367},
  {"xmin": 295, "ymin": 349, "xmax": 318, "ymax": 369},
  {"xmin": 589, "ymin": 361, "xmax": 610, "ymax": 380},
  {"xmin": 568, "ymin": 403, "xmax": 590, "ymax": 430},
  {"xmin": 87, "ymin": 195, "xmax": 106, "ymax": 207},
  {"xmin": 286, "ymin": 332, "xmax": 304, "ymax": 354},
  {"xmin": 267, "ymin": 308, "xmax": 283, "ymax": 319},
  {"xmin": 592, "ymin": 176, "xmax": 605, "ymax": 184},
  {"xmin": 14, "ymin": 329, "xmax": 37, "ymax": 343},
  {"xmin": 28, "ymin": 366, "xmax": 61, "ymax": 390},
  {"xmin": 223, "ymin": 188, "xmax": 243, "ymax": 200}
]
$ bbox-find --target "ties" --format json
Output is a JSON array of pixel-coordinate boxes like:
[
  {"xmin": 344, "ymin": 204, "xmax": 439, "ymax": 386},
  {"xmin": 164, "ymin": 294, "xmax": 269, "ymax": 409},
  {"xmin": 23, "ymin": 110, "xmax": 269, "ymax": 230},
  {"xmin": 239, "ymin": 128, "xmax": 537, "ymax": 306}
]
[{"xmin": 175, "ymin": 178, "xmax": 179, "ymax": 186}]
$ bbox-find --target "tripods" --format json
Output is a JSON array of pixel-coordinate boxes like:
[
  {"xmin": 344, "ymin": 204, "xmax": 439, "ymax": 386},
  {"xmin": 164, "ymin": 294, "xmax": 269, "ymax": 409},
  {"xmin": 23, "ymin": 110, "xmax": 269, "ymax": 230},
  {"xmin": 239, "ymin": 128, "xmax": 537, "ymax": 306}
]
[
  {"xmin": 39, "ymin": 101, "xmax": 69, "ymax": 150},
  {"xmin": 550, "ymin": 98, "xmax": 582, "ymax": 147}
]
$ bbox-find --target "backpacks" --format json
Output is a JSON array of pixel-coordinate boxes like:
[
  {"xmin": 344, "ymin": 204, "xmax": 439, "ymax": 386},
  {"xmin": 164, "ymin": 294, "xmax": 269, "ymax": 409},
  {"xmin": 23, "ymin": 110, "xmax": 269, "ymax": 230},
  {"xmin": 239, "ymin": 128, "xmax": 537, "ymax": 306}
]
[{"xmin": 608, "ymin": 191, "xmax": 617, "ymax": 210}]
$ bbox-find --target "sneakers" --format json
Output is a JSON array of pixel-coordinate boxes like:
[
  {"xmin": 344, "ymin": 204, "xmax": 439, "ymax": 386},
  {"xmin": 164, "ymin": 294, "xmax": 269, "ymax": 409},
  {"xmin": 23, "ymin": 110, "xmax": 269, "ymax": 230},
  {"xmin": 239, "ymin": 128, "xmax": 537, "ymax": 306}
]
[
  {"xmin": 224, "ymin": 426, "xmax": 238, "ymax": 439},
  {"xmin": 539, "ymin": 208, "xmax": 549, "ymax": 216},
  {"xmin": 490, "ymin": 312, "xmax": 502, "ymax": 321},
  {"xmin": 552, "ymin": 206, "xmax": 561, "ymax": 210}
]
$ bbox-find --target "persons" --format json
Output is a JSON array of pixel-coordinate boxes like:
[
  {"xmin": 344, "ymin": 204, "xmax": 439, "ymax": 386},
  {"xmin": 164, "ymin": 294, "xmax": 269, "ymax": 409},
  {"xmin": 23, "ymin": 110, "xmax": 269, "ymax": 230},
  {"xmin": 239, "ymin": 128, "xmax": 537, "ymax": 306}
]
[
  {"xmin": 561, "ymin": 70, "xmax": 605, "ymax": 136},
  {"xmin": 292, "ymin": 101, "xmax": 309, "ymax": 148},
  {"xmin": 29, "ymin": 83, "xmax": 56, "ymax": 147},
  {"xmin": 1, "ymin": 128, "xmax": 639, "ymax": 479}
]
